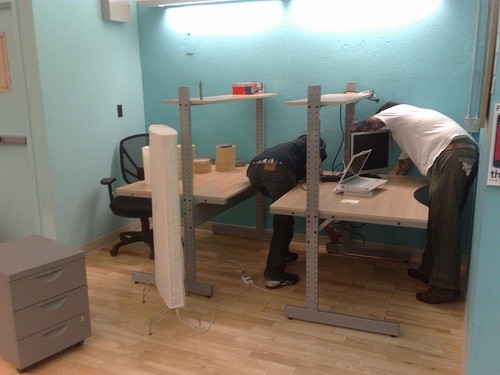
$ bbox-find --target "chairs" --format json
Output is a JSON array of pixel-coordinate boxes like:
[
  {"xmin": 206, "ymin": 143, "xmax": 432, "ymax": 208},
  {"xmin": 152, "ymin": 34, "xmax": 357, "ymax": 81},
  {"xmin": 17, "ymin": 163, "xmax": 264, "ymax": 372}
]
[{"xmin": 101, "ymin": 134, "xmax": 156, "ymax": 259}]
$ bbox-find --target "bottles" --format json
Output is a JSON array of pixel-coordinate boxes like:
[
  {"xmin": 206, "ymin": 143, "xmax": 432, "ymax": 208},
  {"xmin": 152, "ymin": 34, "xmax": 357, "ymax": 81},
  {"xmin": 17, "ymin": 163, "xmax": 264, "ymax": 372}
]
[{"xmin": 232, "ymin": 82, "xmax": 264, "ymax": 95}]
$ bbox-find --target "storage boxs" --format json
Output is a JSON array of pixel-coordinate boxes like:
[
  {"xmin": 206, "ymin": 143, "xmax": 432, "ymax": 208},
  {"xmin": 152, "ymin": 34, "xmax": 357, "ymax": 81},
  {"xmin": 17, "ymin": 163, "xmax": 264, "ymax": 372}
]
[{"xmin": 231, "ymin": 82, "xmax": 263, "ymax": 95}]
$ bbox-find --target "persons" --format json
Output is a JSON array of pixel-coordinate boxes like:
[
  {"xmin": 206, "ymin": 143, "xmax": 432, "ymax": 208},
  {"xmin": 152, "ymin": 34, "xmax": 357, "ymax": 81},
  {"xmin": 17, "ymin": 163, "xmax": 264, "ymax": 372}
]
[
  {"xmin": 246, "ymin": 133, "xmax": 327, "ymax": 290},
  {"xmin": 352, "ymin": 101, "xmax": 480, "ymax": 303}
]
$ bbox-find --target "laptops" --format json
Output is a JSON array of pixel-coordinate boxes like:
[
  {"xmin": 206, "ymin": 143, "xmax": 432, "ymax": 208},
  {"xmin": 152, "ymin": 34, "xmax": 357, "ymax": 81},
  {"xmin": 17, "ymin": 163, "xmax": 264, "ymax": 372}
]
[{"xmin": 334, "ymin": 149, "xmax": 388, "ymax": 193}]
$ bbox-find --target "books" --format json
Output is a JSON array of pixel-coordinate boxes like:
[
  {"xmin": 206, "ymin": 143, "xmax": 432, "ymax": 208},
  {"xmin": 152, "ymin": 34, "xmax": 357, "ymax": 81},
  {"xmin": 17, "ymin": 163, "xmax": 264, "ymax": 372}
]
[{"xmin": 344, "ymin": 191, "xmax": 373, "ymax": 197}]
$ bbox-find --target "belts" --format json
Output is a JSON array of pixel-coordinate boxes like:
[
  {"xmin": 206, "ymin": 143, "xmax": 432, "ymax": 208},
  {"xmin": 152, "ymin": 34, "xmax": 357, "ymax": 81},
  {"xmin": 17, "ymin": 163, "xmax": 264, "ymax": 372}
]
[
  {"xmin": 254, "ymin": 158, "xmax": 285, "ymax": 165},
  {"xmin": 446, "ymin": 142, "xmax": 477, "ymax": 151}
]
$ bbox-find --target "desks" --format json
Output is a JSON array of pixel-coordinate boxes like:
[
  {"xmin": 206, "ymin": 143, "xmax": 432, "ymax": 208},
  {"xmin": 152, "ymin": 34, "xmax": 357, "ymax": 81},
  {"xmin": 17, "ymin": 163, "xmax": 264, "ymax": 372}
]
[
  {"xmin": 116, "ymin": 163, "xmax": 253, "ymax": 298},
  {"xmin": 269, "ymin": 171, "xmax": 430, "ymax": 337}
]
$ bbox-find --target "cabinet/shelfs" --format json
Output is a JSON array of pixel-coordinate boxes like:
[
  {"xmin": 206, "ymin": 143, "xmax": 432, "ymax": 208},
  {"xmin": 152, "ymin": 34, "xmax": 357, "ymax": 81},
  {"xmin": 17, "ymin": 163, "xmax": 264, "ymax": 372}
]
[{"xmin": 0, "ymin": 235, "xmax": 90, "ymax": 375}]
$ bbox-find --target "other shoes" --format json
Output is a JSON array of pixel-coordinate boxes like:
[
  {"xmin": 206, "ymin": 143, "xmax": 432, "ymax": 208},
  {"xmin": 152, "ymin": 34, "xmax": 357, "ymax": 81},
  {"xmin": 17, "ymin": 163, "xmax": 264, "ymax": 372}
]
[
  {"xmin": 279, "ymin": 250, "xmax": 298, "ymax": 262},
  {"xmin": 416, "ymin": 286, "xmax": 465, "ymax": 304},
  {"xmin": 265, "ymin": 273, "xmax": 300, "ymax": 290},
  {"xmin": 407, "ymin": 267, "xmax": 434, "ymax": 284}
]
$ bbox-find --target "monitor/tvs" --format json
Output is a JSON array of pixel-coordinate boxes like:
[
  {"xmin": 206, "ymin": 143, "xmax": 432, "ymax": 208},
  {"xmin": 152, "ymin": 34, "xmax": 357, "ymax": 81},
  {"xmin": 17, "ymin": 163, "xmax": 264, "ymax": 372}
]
[{"xmin": 352, "ymin": 130, "xmax": 391, "ymax": 174}]
